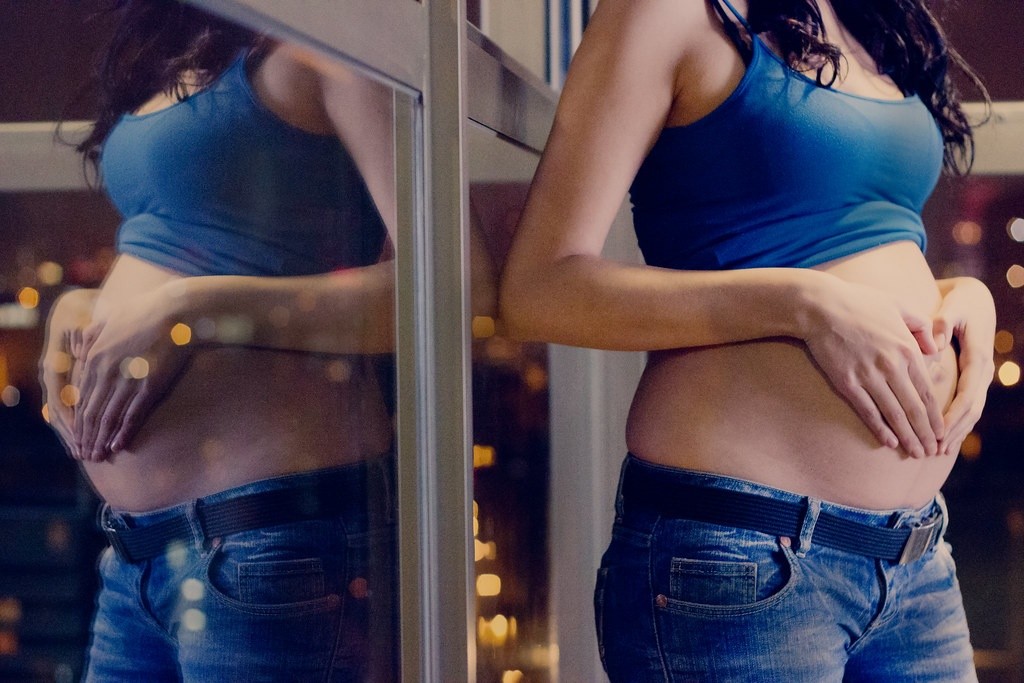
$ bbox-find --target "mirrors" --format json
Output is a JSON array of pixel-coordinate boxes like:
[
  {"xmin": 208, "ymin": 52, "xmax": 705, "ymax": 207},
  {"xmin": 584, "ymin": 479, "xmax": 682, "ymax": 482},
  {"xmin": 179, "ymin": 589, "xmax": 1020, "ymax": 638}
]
[{"xmin": 0, "ymin": 0, "xmax": 550, "ymax": 682}]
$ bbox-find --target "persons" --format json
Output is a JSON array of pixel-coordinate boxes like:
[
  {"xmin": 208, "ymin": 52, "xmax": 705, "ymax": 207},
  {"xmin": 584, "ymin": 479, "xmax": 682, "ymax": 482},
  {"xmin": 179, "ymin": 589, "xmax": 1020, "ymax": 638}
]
[
  {"xmin": 36, "ymin": 0, "xmax": 399, "ymax": 683},
  {"xmin": 495, "ymin": 0, "xmax": 999, "ymax": 683}
]
